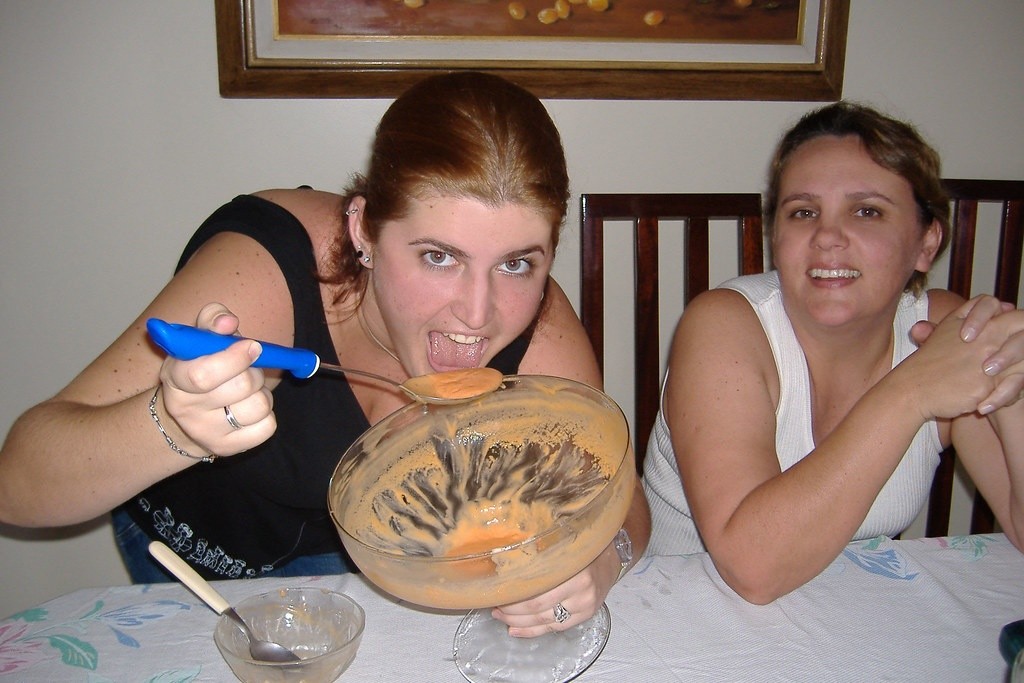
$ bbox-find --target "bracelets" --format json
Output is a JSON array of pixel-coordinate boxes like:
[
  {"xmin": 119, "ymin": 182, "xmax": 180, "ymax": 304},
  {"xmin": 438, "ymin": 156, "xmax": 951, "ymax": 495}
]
[{"xmin": 149, "ymin": 385, "xmax": 218, "ymax": 463}]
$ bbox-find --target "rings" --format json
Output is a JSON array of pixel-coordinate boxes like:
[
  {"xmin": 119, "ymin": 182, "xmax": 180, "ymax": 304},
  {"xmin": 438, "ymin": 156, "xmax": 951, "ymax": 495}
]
[
  {"xmin": 552, "ymin": 603, "xmax": 572, "ymax": 624},
  {"xmin": 224, "ymin": 405, "xmax": 243, "ymax": 430}
]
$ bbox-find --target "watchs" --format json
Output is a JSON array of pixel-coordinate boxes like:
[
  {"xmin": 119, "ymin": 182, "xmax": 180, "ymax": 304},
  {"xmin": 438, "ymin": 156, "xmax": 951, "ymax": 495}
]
[{"xmin": 613, "ymin": 527, "xmax": 634, "ymax": 584}]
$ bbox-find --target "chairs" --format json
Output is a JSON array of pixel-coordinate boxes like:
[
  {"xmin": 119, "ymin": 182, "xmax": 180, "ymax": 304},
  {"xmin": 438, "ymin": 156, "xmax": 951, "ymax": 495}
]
[
  {"xmin": 894, "ymin": 177, "xmax": 1024, "ymax": 541},
  {"xmin": 580, "ymin": 192, "xmax": 763, "ymax": 478}
]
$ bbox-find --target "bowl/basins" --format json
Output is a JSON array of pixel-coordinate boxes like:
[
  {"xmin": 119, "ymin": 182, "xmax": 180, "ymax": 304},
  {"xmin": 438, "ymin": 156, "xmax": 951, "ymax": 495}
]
[{"xmin": 213, "ymin": 588, "xmax": 366, "ymax": 683}]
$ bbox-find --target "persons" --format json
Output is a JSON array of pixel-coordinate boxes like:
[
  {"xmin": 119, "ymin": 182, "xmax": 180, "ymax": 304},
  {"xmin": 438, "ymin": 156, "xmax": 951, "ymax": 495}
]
[
  {"xmin": 642, "ymin": 98, "xmax": 1024, "ymax": 606},
  {"xmin": 0, "ymin": 73, "xmax": 653, "ymax": 638}
]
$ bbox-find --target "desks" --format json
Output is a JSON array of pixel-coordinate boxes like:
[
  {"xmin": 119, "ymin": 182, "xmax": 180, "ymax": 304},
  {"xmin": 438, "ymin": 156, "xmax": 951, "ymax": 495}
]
[{"xmin": 0, "ymin": 533, "xmax": 1024, "ymax": 683}]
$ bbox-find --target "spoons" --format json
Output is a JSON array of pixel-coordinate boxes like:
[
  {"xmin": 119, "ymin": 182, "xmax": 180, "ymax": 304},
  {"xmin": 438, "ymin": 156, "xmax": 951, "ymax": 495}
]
[{"xmin": 149, "ymin": 541, "xmax": 302, "ymax": 670}]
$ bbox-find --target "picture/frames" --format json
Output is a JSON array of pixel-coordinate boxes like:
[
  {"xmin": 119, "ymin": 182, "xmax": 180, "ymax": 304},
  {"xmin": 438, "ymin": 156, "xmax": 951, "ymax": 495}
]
[{"xmin": 214, "ymin": 0, "xmax": 850, "ymax": 102}]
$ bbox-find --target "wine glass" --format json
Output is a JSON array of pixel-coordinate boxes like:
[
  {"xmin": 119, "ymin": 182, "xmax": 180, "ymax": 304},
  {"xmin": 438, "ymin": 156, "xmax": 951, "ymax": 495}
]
[{"xmin": 327, "ymin": 375, "xmax": 636, "ymax": 683}]
